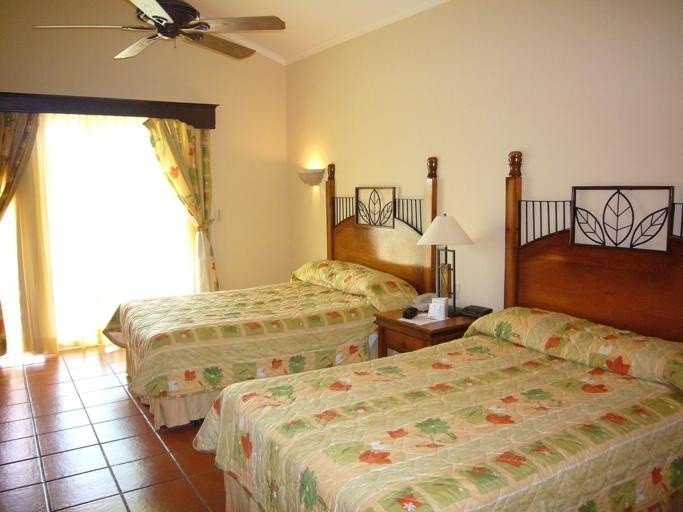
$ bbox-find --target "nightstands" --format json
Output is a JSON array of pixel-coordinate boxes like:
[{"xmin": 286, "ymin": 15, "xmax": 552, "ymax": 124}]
[{"xmin": 367, "ymin": 301, "xmax": 484, "ymax": 358}]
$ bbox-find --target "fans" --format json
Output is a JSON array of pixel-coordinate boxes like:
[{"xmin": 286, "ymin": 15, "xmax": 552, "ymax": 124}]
[{"xmin": 29, "ymin": 0, "xmax": 288, "ymax": 62}]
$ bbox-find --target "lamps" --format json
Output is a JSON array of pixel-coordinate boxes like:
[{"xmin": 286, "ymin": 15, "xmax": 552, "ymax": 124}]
[
  {"xmin": 293, "ymin": 163, "xmax": 327, "ymax": 189},
  {"xmin": 414, "ymin": 210, "xmax": 474, "ymax": 317}
]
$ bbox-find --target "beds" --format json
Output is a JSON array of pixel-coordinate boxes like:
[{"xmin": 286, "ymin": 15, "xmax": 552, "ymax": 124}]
[
  {"xmin": 102, "ymin": 154, "xmax": 444, "ymax": 431},
  {"xmin": 185, "ymin": 145, "xmax": 682, "ymax": 512}
]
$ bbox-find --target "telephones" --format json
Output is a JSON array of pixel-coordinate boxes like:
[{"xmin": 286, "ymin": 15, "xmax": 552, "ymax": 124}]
[{"xmin": 462, "ymin": 305, "xmax": 493, "ymax": 318}]
